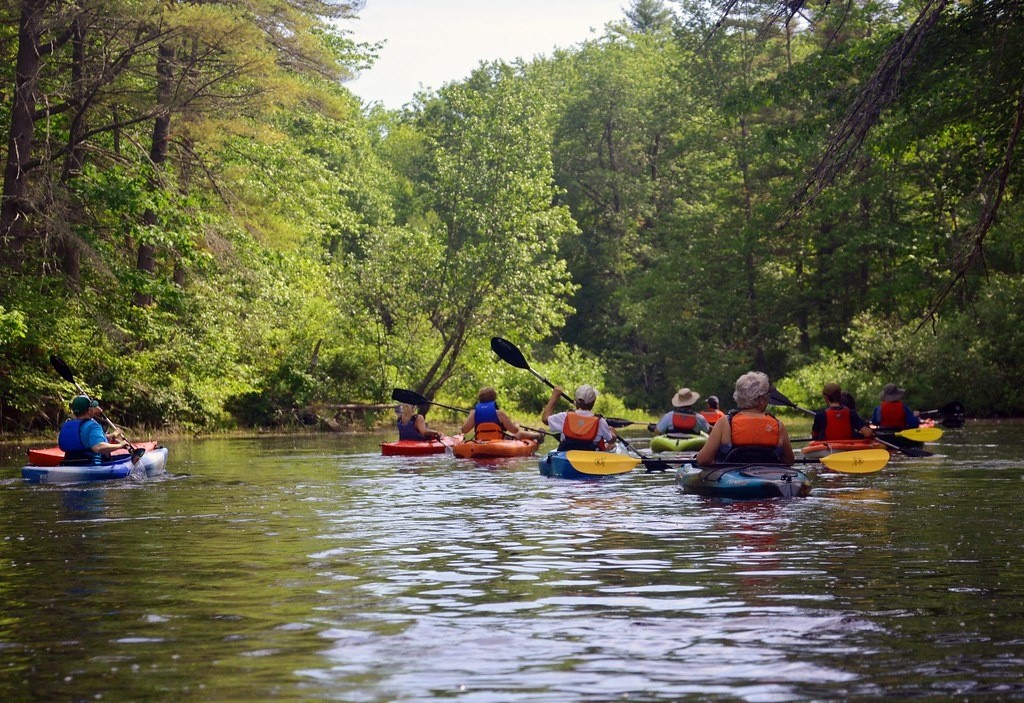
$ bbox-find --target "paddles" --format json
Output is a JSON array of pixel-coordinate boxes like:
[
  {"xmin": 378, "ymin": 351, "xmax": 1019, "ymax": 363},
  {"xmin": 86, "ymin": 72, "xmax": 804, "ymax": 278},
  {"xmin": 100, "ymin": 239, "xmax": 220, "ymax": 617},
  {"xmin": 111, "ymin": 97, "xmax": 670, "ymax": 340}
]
[
  {"xmin": 603, "ymin": 416, "xmax": 654, "ymax": 429},
  {"xmin": 489, "ymin": 334, "xmax": 672, "ymax": 473},
  {"xmin": 565, "ymin": 449, "xmax": 891, "ymax": 475},
  {"xmin": 391, "ymin": 387, "xmax": 561, "ymax": 443},
  {"xmin": 394, "ymin": 405, "xmax": 454, "ymax": 454},
  {"xmin": 917, "ymin": 401, "xmax": 965, "ymax": 414},
  {"xmin": 789, "ymin": 427, "xmax": 944, "ymax": 443},
  {"xmin": 766, "ymin": 388, "xmax": 934, "ymax": 457},
  {"xmin": 920, "ymin": 416, "xmax": 966, "ymax": 428}
]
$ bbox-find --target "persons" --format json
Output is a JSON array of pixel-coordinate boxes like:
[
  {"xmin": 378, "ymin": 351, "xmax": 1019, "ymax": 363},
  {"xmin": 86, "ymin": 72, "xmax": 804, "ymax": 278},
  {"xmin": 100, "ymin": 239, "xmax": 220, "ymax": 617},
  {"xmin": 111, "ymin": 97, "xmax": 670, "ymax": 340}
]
[
  {"xmin": 872, "ymin": 384, "xmax": 919, "ymax": 430},
  {"xmin": 397, "ymin": 404, "xmax": 441, "ymax": 440},
  {"xmin": 542, "ymin": 385, "xmax": 617, "ymax": 453},
  {"xmin": 59, "ymin": 396, "xmax": 129, "ymax": 459},
  {"xmin": 654, "ymin": 388, "xmax": 710, "ymax": 439},
  {"xmin": 461, "ymin": 388, "xmax": 546, "ymax": 443},
  {"xmin": 696, "ymin": 372, "xmax": 794, "ymax": 466},
  {"xmin": 811, "ymin": 383, "xmax": 873, "ymax": 441},
  {"xmin": 700, "ymin": 396, "xmax": 724, "ymax": 426}
]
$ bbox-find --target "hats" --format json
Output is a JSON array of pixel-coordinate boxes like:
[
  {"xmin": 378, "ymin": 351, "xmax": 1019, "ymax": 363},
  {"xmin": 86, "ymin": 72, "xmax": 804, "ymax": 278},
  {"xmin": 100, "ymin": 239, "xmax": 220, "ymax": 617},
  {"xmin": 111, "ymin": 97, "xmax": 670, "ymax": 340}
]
[
  {"xmin": 574, "ymin": 383, "xmax": 600, "ymax": 404},
  {"xmin": 705, "ymin": 396, "xmax": 719, "ymax": 404},
  {"xmin": 672, "ymin": 388, "xmax": 700, "ymax": 407},
  {"xmin": 879, "ymin": 383, "xmax": 905, "ymax": 402},
  {"xmin": 70, "ymin": 395, "xmax": 99, "ymax": 413}
]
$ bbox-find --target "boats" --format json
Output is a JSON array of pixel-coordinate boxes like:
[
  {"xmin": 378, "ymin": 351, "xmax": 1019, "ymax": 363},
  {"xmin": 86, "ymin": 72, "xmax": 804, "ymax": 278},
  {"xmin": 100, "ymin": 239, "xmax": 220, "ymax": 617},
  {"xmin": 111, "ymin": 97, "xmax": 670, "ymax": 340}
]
[
  {"xmin": 801, "ymin": 436, "xmax": 891, "ymax": 463},
  {"xmin": 675, "ymin": 455, "xmax": 811, "ymax": 498},
  {"xmin": 536, "ymin": 441, "xmax": 632, "ymax": 478},
  {"xmin": 25, "ymin": 439, "xmax": 161, "ymax": 465},
  {"xmin": 649, "ymin": 430, "xmax": 712, "ymax": 454},
  {"xmin": 865, "ymin": 418, "xmax": 941, "ymax": 437},
  {"xmin": 20, "ymin": 445, "xmax": 169, "ymax": 486},
  {"xmin": 378, "ymin": 431, "xmax": 466, "ymax": 457},
  {"xmin": 457, "ymin": 437, "xmax": 540, "ymax": 462}
]
